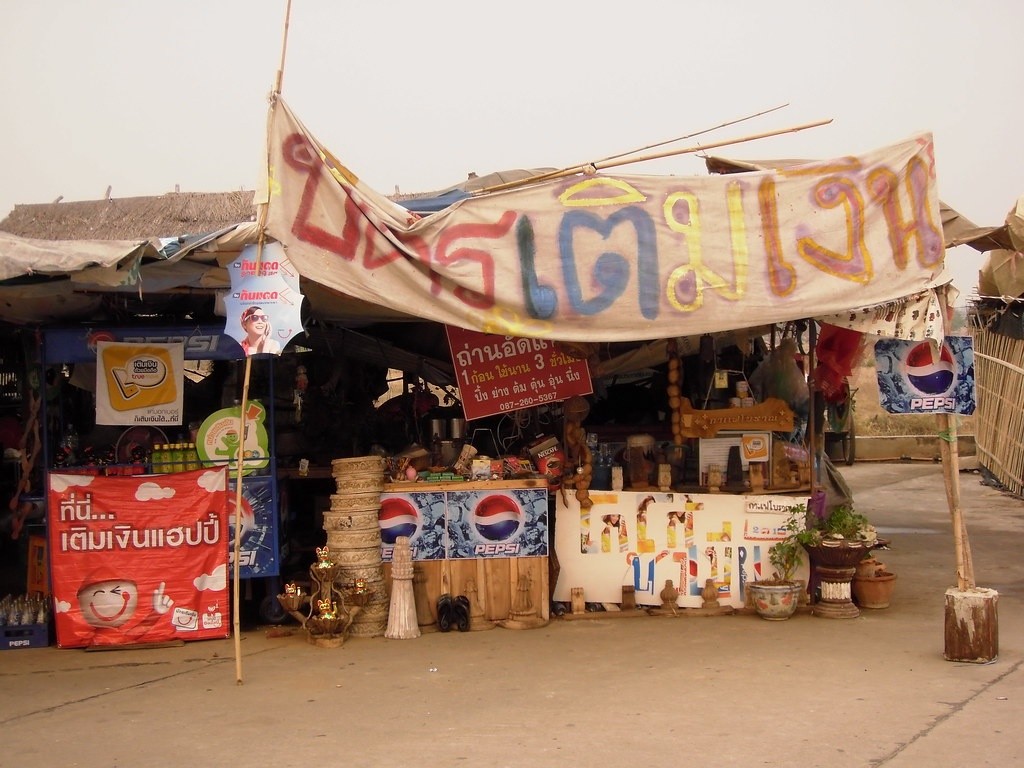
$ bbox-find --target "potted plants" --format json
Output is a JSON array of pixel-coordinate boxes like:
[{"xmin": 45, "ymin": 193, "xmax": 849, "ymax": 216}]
[{"xmin": 768, "ymin": 503, "xmax": 891, "ymax": 619}]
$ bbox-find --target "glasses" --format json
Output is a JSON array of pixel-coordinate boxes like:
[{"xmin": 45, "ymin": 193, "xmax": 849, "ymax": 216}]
[{"xmin": 244, "ymin": 314, "xmax": 270, "ymax": 322}]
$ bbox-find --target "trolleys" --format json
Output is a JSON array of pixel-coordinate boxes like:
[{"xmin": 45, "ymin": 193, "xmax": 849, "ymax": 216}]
[{"xmin": 792, "ymin": 387, "xmax": 858, "ymax": 465}]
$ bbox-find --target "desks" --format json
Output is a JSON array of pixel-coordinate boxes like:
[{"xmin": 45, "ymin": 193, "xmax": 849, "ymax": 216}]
[{"xmin": 275, "ymin": 464, "xmax": 332, "ymax": 585}]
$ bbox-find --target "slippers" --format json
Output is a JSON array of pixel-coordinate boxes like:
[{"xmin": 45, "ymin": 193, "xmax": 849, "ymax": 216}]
[{"xmin": 437, "ymin": 594, "xmax": 471, "ymax": 632}]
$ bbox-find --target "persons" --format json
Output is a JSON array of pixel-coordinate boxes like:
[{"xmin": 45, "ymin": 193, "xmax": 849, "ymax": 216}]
[
  {"xmin": 378, "ymin": 392, "xmax": 439, "ymax": 422},
  {"xmin": 240, "ymin": 306, "xmax": 281, "ymax": 356}
]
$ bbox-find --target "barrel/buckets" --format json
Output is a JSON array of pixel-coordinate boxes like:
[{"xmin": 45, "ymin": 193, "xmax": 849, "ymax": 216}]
[{"xmin": 728, "ymin": 381, "xmax": 753, "ymax": 409}]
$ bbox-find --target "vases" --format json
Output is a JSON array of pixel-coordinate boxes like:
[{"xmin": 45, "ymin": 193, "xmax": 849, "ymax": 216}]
[
  {"xmin": 852, "ymin": 556, "xmax": 897, "ymax": 609},
  {"xmin": 747, "ymin": 580, "xmax": 801, "ymax": 621}
]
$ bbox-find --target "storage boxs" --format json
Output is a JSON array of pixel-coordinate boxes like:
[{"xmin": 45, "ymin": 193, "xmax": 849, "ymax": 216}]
[
  {"xmin": 315, "ymin": 494, "xmax": 330, "ymax": 528},
  {"xmin": 26, "ymin": 532, "xmax": 48, "ymax": 592},
  {"xmin": 0, "ymin": 608, "xmax": 53, "ymax": 650},
  {"xmin": 529, "ymin": 433, "xmax": 567, "ymax": 479}
]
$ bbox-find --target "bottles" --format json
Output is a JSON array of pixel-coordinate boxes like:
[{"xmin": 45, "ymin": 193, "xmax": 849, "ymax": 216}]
[
  {"xmin": 151, "ymin": 442, "xmax": 205, "ymax": 475},
  {"xmin": 0, "ymin": 591, "xmax": 52, "ymax": 626}
]
